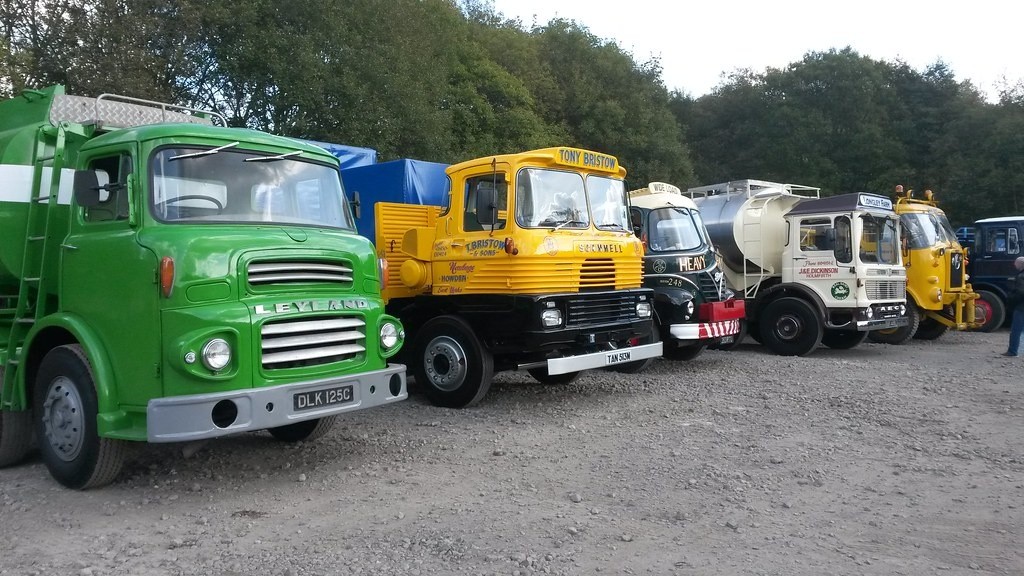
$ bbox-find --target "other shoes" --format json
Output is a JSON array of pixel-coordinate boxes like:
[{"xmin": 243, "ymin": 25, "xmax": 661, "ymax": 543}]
[{"xmin": 996, "ymin": 351, "xmax": 1019, "ymax": 358}]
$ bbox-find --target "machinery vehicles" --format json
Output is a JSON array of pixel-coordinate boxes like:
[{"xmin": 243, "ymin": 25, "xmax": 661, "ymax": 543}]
[{"xmin": 954, "ymin": 215, "xmax": 1024, "ymax": 333}]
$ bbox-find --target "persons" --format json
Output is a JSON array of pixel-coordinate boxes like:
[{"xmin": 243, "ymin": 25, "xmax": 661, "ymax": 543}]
[{"xmin": 1001, "ymin": 257, "xmax": 1024, "ymax": 358}]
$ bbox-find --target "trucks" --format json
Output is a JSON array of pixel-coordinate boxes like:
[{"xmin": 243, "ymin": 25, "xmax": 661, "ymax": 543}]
[
  {"xmin": 273, "ymin": 136, "xmax": 664, "ymax": 410},
  {"xmin": 600, "ymin": 181, "xmax": 746, "ymax": 375},
  {"xmin": 801, "ymin": 185, "xmax": 986, "ymax": 346}
]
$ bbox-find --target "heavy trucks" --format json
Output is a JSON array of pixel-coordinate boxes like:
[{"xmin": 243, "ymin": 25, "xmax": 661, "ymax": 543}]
[
  {"xmin": 681, "ymin": 178, "xmax": 909, "ymax": 359},
  {"xmin": 0, "ymin": 81, "xmax": 408, "ymax": 491}
]
